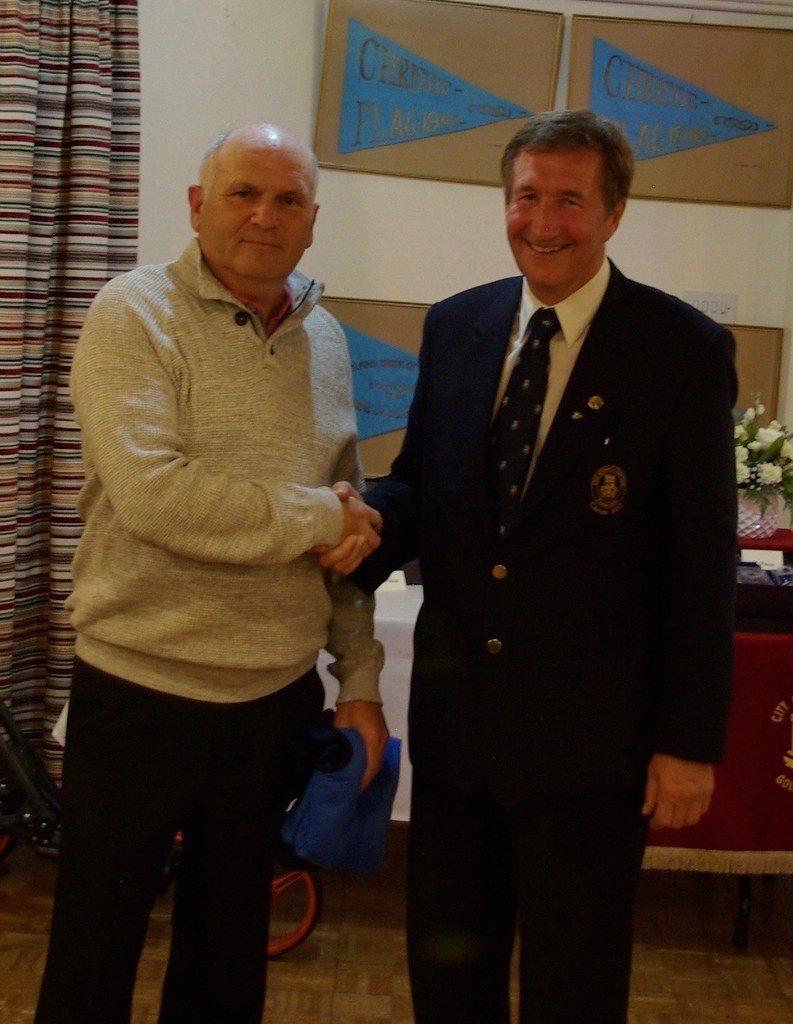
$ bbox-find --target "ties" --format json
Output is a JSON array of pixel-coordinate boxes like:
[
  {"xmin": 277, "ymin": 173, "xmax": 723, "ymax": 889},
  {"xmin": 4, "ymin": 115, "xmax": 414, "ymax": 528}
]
[{"xmin": 490, "ymin": 307, "xmax": 560, "ymax": 507}]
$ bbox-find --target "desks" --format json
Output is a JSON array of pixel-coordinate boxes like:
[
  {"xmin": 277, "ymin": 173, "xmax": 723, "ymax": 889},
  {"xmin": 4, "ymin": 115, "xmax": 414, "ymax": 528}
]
[{"xmin": 639, "ymin": 632, "xmax": 793, "ymax": 950}]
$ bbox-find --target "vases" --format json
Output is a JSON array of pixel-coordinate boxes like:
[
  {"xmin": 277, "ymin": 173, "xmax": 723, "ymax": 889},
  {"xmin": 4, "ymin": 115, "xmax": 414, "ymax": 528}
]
[{"xmin": 734, "ymin": 487, "xmax": 781, "ymax": 539}]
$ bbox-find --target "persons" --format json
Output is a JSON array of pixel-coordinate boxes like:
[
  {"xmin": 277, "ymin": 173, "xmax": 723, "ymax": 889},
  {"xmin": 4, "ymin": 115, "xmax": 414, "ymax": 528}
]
[
  {"xmin": 316, "ymin": 109, "xmax": 742, "ymax": 1024},
  {"xmin": 32, "ymin": 122, "xmax": 384, "ymax": 1024}
]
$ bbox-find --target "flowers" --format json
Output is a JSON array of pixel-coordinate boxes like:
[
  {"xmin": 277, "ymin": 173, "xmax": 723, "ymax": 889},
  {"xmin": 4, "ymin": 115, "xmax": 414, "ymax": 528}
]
[{"xmin": 730, "ymin": 391, "xmax": 793, "ymax": 518}]
